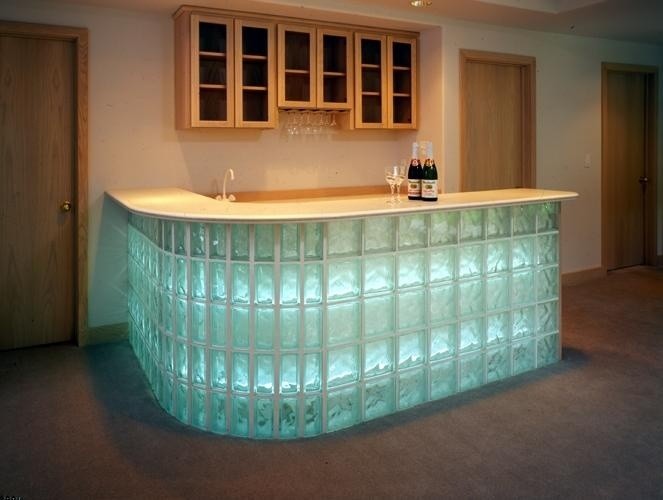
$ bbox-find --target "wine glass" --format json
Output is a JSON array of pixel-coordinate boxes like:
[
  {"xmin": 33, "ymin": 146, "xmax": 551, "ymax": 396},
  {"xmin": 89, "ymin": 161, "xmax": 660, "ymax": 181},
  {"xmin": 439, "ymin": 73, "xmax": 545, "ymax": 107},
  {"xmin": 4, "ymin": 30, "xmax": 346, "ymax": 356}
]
[
  {"xmin": 392, "ymin": 159, "xmax": 407, "ymax": 204},
  {"xmin": 281, "ymin": 110, "xmax": 342, "ymax": 144},
  {"xmin": 384, "ymin": 166, "xmax": 400, "ymax": 204}
]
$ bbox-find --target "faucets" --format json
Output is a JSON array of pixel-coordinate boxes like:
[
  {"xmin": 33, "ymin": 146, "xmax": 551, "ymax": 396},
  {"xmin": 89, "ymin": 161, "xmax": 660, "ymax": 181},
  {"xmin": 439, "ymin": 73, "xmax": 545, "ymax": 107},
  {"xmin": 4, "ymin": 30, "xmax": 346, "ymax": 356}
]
[{"xmin": 222, "ymin": 168, "xmax": 234, "ymax": 199}]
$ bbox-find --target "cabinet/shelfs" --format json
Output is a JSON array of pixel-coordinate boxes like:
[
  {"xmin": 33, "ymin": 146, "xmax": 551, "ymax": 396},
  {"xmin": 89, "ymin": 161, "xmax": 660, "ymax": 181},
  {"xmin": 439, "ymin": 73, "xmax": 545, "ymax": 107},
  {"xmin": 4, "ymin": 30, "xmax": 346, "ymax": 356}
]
[
  {"xmin": 172, "ymin": 4, "xmax": 278, "ymax": 128},
  {"xmin": 278, "ymin": 15, "xmax": 354, "ymax": 109},
  {"xmin": 338, "ymin": 26, "xmax": 418, "ymax": 130}
]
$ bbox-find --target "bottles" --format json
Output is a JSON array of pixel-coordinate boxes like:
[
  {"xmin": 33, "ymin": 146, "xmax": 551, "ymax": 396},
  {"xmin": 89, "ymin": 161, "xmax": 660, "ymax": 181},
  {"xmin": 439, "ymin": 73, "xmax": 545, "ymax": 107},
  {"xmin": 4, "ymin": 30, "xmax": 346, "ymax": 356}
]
[
  {"xmin": 407, "ymin": 142, "xmax": 423, "ymax": 200},
  {"xmin": 421, "ymin": 141, "xmax": 438, "ymax": 201}
]
